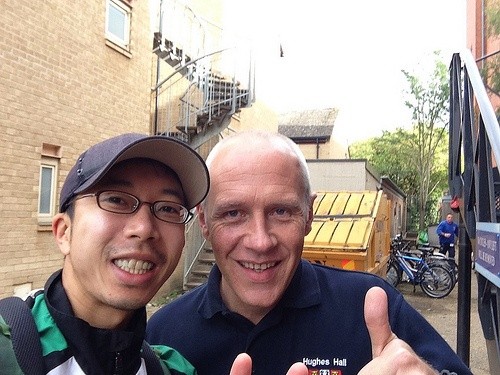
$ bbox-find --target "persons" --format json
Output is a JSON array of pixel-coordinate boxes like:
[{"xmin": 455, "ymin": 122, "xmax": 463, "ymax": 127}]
[
  {"xmin": 147, "ymin": 131, "xmax": 473, "ymax": 375},
  {"xmin": 435, "ymin": 214, "xmax": 458, "ymax": 258},
  {"xmin": 0, "ymin": 132, "xmax": 210, "ymax": 375}
]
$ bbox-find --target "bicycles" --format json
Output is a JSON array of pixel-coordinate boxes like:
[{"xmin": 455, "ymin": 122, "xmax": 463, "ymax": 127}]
[{"xmin": 385, "ymin": 231, "xmax": 459, "ymax": 298}]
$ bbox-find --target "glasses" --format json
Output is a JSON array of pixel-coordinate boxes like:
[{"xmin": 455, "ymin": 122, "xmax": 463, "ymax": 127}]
[{"xmin": 66, "ymin": 190, "xmax": 195, "ymax": 224}]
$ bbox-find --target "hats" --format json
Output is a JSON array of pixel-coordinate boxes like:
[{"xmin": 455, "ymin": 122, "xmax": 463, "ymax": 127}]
[{"xmin": 59, "ymin": 133, "xmax": 210, "ymax": 212}]
